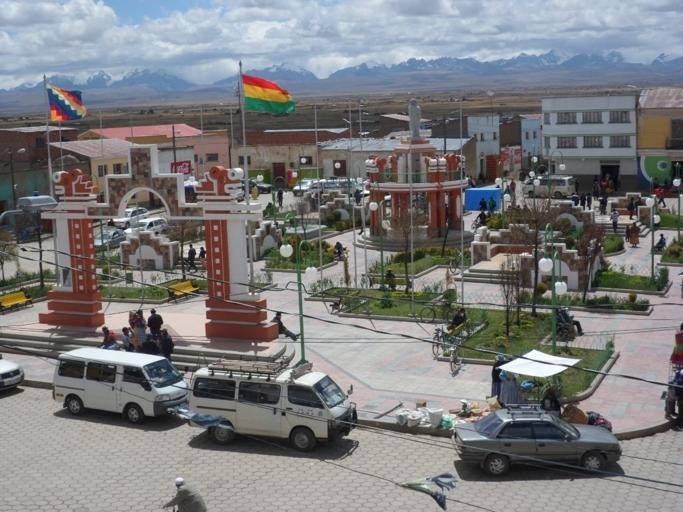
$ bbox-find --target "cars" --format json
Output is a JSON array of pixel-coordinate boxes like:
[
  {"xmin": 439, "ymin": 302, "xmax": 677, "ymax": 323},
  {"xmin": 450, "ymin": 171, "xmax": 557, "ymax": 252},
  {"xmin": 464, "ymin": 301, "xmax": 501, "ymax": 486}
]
[
  {"xmin": 125, "ymin": 217, "xmax": 169, "ymax": 235},
  {"xmin": 93, "ymin": 228, "xmax": 125, "ymax": 249},
  {"xmin": 452, "ymin": 408, "xmax": 621, "ymax": 475},
  {"xmin": 0, "ymin": 354, "xmax": 23, "ymax": 392}
]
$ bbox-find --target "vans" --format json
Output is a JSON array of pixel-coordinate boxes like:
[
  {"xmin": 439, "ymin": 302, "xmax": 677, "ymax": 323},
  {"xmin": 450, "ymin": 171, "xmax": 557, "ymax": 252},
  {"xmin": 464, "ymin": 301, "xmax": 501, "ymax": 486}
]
[
  {"xmin": 52, "ymin": 346, "xmax": 188, "ymax": 424},
  {"xmin": 523, "ymin": 174, "xmax": 577, "ymax": 198},
  {"xmin": 186, "ymin": 361, "xmax": 357, "ymax": 449}
]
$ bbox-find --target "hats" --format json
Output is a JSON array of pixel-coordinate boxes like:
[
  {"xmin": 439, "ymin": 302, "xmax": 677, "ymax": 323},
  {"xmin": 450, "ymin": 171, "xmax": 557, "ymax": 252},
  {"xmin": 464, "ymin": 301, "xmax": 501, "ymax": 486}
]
[{"xmin": 175, "ymin": 477, "xmax": 184, "ymax": 485}]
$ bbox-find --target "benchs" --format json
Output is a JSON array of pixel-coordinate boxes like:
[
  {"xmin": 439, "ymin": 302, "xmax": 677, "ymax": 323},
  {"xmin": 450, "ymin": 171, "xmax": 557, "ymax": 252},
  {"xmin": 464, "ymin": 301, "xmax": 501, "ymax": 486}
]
[
  {"xmin": 0, "ymin": 291, "xmax": 33, "ymax": 315},
  {"xmin": 448, "ymin": 320, "xmax": 465, "ymax": 336},
  {"xmin": 167, "ymin": 280, "xmax": 199, "ymax": 303},
  {"xmin": 366, "ymin": 273, "xmax": 412, "ymax": 294},
  {"xmin": 654, "ymin": 242, "xmax": 668, "ymax": 253}
]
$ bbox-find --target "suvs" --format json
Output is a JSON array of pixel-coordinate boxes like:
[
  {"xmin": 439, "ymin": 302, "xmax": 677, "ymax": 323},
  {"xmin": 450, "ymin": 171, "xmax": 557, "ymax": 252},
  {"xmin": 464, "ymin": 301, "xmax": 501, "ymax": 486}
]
[
  {"xmin": 112, "ymin": 207, "xmax": 148, "ymax": 228},
  {"xmin": 293, "ymin": 179, "xmax": 312, "ymax": 194},
  {"xmin": 243, "ymin": 178, "xmax": 271, "ymax": 194}
]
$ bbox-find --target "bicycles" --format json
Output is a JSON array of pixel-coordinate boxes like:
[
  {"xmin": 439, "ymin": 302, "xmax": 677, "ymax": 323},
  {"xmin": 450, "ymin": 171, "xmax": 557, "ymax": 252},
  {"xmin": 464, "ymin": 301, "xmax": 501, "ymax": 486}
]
[
  {"xmin": 471, "ymin": 217, "xmax": 492, "ymax": 233},
  {"xmin": 420, "ymin": 300, "xmax": 459, "ymax": 323},
  {"xmin": 448, "ymin": 248, "xmax": 469, "ymax": 273},
  {"xmin": 432, "ymin": 327, "xmax": 465, "ymax": 373}
]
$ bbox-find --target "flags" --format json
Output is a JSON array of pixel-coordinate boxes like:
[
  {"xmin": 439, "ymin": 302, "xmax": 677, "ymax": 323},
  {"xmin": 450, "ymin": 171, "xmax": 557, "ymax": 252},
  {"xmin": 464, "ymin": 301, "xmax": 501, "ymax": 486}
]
[
  {"xmin": 45, "ymin": 83, "xmax": 88, "ymax": 122},
  {"xmin": 241, "ymin": 74, "xmax": 295, "ymax": 116}
]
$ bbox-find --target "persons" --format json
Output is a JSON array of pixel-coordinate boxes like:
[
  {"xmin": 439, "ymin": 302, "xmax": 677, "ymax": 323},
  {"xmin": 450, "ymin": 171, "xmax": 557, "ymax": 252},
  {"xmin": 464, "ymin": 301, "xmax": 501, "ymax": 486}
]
[
  {"xmin": 99, "ymin": 307, "xmax": 173, "ymax": 362},
  {"xmin": 198, "ymin": 246, "xmax": 206, "ymax": 260},
  {"xmin": 561, "ymin": 304, "xmax": 584, "ymax": 336},
  {"xmin": 586, "ymin": 193, "xmax": 592, "ymax": 210},
  {"xmin": 625, "ymin": 196, "xmax": 635, "ymax": 220},
  {"xmin": 579, "ymin": 192, "xmax": 586, "ymax": 210},
  {"xmin": 609, "ymin": 208, "xmax": 619, "ymax": 233},
  {"xmin": 477, "ymin": 208, "xmax": 485, "ymax": 225},
  {"xmin": 629, "ymin": 221, "xmax": 639, "ymax": 247},
  {"xmin": 160, "ymin": 476, "xmax": 207, "ymax": 512},
  {"xmin": 571, "ymin": 192, "xmax": 579, "ymax": 206},
  {"xmin": 186, "ymin": 244, "xmax": 197, "ymax": 272},
  {"xmin": 465, "ymin": 172, "xmax": 527, "ymax": 196},
  {"xmin": 450, "ymin": 307, "xmax": 465, "ymax": 326},
  {"xmin": 598, "ymin": 195, "xmax": 608, "ymax": 215},
  {"xmin": 655, "ymin": 232, "xmax": 664, "ymax": 247},
  {"xmin": 656, "ymin": 191, "xmax": 666, "ymax": 208},
  {"xmin": 270, "ymin": 311, "xmax": 297, "ymax": 341},
  {"xmin": 486, "ymin": 196, "xmax": 495, "ymax": 213},
  {"xmin": 384, "ymin": 267, "xmax": 396, "ymax": 291},
  {"xmin": 353, "ymin": 188, "xmax": 361, "ymax": 205},
  {"xmin": 479, "ymin": 197, "xmax": 486, "ymax": 211},
  {"xmin": 634, "ymin": 196, "xmax": 643, "ymax": 217},
  {"xmin": 624, "ymin": 224, "xmax": 630, "ymax": 242},
  {"xmin": 540, "ymin": 387, "xmax": 560, "ymax": 412},
  {"xmin": 572, "ymin": 171, "xmax": 618, "ymax": 200},
  {"xmin": 276, "ymin": 187, "xmax": 283, "ymax": 206},
  {"xmin": 491, "ymin": 353, "xmax": 505, "ymax": 382}
]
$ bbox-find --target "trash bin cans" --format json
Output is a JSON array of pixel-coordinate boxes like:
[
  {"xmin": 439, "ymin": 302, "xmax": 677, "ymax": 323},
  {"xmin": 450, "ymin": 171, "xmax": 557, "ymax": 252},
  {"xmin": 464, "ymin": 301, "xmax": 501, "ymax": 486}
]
[
  {"xmin": 446, "ymin": 289, "xmax": 456, "ymax": 302},
  {"xmin": 125, "ymin": 273, "xmax": 132, "ymax": 283}
]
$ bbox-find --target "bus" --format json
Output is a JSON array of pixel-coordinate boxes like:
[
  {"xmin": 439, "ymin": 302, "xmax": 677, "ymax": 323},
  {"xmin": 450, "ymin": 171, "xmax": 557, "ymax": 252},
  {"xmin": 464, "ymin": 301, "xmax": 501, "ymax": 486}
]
[{"xmin": 311, "ymin": 177, "xmax": 356, "ymax": 195}]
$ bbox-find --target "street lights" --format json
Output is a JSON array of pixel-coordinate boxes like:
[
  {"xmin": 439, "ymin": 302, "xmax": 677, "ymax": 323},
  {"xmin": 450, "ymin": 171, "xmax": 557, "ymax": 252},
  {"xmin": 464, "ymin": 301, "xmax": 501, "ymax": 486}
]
[
  {"xmin": 369, "ymin": 192, "xmax": 392, "ymax": 289},
  {"xmin": 646, "ymin": 194, "xmax": 661, "ymax": 279},
  {"xmin": 280, "ymin": 212, "xmax": 313, "ymax": 359},
  {"xmin": 529, "ymin": 149, "xmax": 565, "ymax": 208},
  {"xmin": 495, "ymin": 159, "xmax": 513, "ymax": 229},
  {"xmin": 537, "ymin": 222, "xmax": 568, "ymax": 354},
  {"xmin": 296, "ymin": 153, "xmax": 308, "ymax": 194},
  {"xmin": 672, "ymin": 162, "xmax": 681, "ymax": 240}
]
